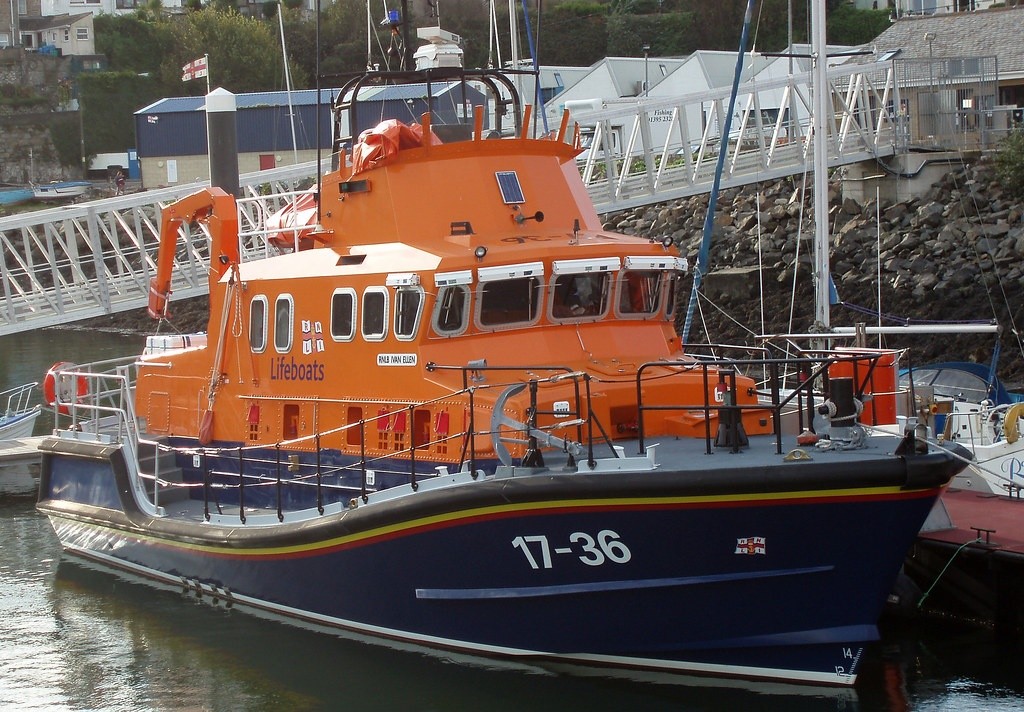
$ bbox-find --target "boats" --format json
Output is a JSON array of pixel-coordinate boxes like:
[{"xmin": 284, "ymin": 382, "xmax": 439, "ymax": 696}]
[{"xmin": 1, "ymin": 1, "xmax": 1024, "ymax": 704}]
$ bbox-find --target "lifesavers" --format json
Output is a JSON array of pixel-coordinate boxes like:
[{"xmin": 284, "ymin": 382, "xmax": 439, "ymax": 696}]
[{"xmin": 43, "ymin": 360, "xmax": 93, "ymax": 415}]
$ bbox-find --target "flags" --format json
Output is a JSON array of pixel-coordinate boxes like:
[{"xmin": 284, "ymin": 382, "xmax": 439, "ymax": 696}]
[{"xmin": 182, "ymin": 57, "xmax": 207, "ymax": 81}]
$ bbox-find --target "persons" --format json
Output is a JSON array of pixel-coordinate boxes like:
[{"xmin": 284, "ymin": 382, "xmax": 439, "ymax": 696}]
[{"xmin": 114, "ymin": 171, "xmax": 124, "ymax": 197}]
